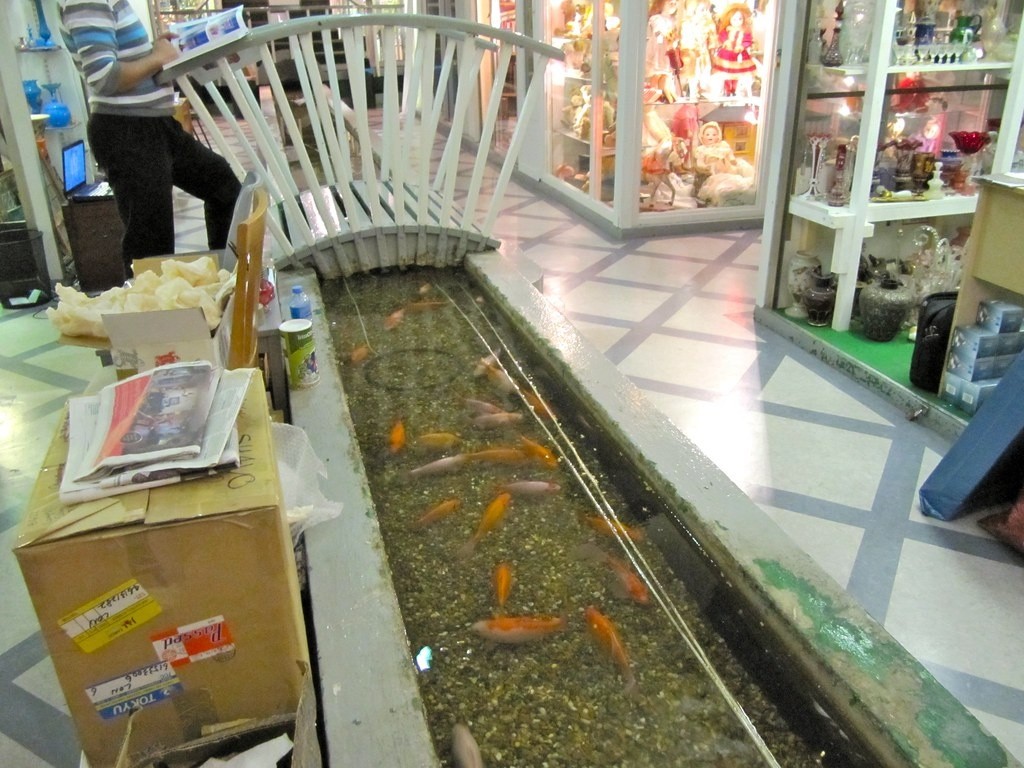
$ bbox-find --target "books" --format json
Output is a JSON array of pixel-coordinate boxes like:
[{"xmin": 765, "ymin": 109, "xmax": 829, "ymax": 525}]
[{"xmin": 162, "ymin": 5, "xmax": 250, "ymax": 70}]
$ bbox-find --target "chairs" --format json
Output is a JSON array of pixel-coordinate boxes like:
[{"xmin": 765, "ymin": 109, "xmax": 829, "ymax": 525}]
[{"xmin": 112, "ymin": 179, "xmax": 268, "ymax": 378}]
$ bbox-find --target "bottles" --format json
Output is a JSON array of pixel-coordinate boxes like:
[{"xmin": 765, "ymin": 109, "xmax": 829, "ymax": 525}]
[{"xmin": 290, "ymin": 286, "xmax": 312, "ymax": 325}]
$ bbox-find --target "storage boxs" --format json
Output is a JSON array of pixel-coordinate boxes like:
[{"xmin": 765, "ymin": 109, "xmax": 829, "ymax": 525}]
[
  {"xmin": 8, "ymin": 365, "xmax": 310, "ymax": 764},
  {"xmin": 116, "ymin": 657, "xmax": 325, "ymax": 766}
]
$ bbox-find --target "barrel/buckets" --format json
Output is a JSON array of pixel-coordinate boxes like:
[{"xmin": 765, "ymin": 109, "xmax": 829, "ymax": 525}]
[{"xmin": 279, "ymin": 319, "xmax": 319, "ymax": 393}]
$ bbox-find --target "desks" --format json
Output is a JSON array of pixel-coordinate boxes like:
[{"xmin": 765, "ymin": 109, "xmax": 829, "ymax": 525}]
[
  {"xmin": 96, "ymin": 267, "xmax": 286, "ymax": 412},
  {"xmin": 936, "ymin": 171, "xmax": 1024, "ymax": 397}
]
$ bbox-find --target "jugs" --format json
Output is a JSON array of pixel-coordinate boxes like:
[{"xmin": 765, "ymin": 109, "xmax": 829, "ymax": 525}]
[{"xmin": 949, "ymin": 15, "xmax": 981, "ymax": 46}]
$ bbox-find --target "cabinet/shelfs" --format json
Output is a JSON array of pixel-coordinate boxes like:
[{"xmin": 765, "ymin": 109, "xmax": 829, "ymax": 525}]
[
  {"xmin": 60, "ymin": 197, "xmax": 131, "ymax": 292},
  {"xmin": 772, "ymin": 0, "xmax": 1024, "ymax": 331},
  {"xmin": 542, "ymin": 0, "xmax": 787, "ymax": 230}
]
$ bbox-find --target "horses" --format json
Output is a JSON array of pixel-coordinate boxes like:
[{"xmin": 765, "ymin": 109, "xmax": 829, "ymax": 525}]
[{"xmin": 641, "ymin": 137, "xmax": 688, "ymax": 208}]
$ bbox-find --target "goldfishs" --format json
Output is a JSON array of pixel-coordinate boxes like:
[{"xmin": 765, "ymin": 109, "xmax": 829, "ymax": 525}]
[
  {"xmin": 419, "ymin": 283, "xmax": 432, "ymax": 295},
  {"xmin": 350, "ymin": 346, "xmax": 368, "ymax": 362},
  {"xmin": 450, "ymin": 389, "xmax": 507, "ymax": 414},
  {"xmin": 491, "ymin": 562, "xmax": 512, "ymax": 609},
  {"xmin": 410, "ymin": 497, "xmax": 461, "ymax": 531},
  {"xmin": 410, "ymin": 453, "xmax": 466, "ymax": 477},
  {"xmin": 586, "ymin": 514, "xmax": 643, "ymax": 541},
  {"xmin": 467, "ymin": 448, "xmax": 525, "ymax": 465},
  {"xmin": 383, "ymin": 307, "xmax": 408, "ymax": 332},
  {"xmin": 470, "ymin": 618, "xmax": 563, "ymax": 645},
  {"xmin": 607, "ymin": 555, "xmax": 651, "ymax": 606},
  {"xmin": 473, "ymin": 348, "xmax": 502, "ymax": 379},
  {"xmin": 518, "ymin": 388, "xmax": 561, "ymax": 421},
  {"xmin": 498, "ymin": 481, "xmax": 563, "ymax": 498},
  {"xmin": 516, "ymin": 435, "xmax": 556, "ymax": 466},
  {"xmin": 407, "ymin": 434, "xmax": 471, "ymax": 451},
  {"xmin": 472, "ymin": 412, "xmax": 521, "ymax": 428},
  {"xmin": 586, "ymin": 608, "xmax": 636, "ymax": 690},
  {"xmin": 387, "ymin": 418, "xmax": 407, "ymax": 455},
  {"xmin": 458, "ymin": 494, "xmax": 512, "ymax": 552},
  {"xmin": 408, "ymin": 301, "xmax": 451, "ymax": 312},
  {"xmin": 479, "ymin": 359, "xmax": 521, "ymax": 395},
  {"xmin": 449, "ymin": 724, "xmax": 485, "ymax": 768}
]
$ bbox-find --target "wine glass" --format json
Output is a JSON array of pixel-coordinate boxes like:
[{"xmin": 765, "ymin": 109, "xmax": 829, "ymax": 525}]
[{"xmin": 895, "ymin": 42, "xmax": 964, "ymax": 66}]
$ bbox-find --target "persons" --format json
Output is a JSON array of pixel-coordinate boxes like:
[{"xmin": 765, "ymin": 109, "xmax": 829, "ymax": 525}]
[
  {"xmin": 57, "ymin": 1, "xmax": 243, "ymax": 279},
  {"xmin": 553, "ymin": 0, "xmax": 760, "ymax": 206}
]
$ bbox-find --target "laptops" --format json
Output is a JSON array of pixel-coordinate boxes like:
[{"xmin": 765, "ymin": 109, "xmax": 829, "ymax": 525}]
[{"xmin": 62, "ymin": 139, "xmax": 114, "ymax": 200}]
